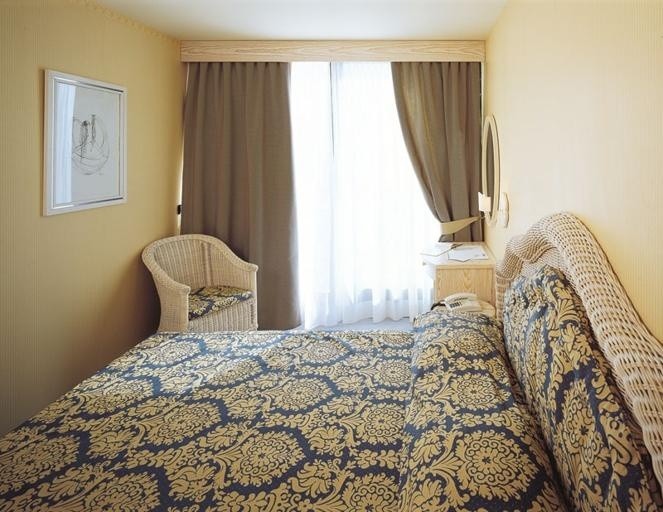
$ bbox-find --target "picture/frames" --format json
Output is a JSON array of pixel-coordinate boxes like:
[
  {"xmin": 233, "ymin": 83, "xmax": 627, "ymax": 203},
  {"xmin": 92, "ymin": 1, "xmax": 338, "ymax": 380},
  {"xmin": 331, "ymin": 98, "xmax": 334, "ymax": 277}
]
[{"xmin": 41, "ymin": 68, "xmax": 128, "ymax": 217}]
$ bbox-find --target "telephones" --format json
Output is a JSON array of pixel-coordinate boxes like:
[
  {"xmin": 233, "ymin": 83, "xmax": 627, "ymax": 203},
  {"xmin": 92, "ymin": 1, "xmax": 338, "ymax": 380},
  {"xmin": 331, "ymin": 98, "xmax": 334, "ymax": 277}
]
[{"xmin": 443, "ymin": 293, "xmax": 482, "ymax": 312}]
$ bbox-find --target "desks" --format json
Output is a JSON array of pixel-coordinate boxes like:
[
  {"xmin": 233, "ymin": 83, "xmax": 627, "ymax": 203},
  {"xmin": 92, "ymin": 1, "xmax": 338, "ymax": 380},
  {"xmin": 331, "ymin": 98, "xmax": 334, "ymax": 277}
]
[
  {"xmin": 424, "ymin": 240, "xmax": 496, "ymax": 313},
  {"xmin": 431, "ymin": 294, "xmax": 497, "ymax": 322}
]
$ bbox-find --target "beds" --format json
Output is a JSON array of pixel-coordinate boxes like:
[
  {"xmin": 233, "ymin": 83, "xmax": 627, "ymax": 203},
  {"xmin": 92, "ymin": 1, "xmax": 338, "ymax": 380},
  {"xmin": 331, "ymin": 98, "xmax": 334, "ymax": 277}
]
[{"xmin": 0, "ymin": 211, "xmax": 663, "ymax": 512}]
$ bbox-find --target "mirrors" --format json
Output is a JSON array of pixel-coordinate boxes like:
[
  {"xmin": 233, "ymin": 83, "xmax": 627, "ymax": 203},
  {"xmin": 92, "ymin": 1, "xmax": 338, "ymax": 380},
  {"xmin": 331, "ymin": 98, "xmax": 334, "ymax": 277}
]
[{"xmin": 479, "ymin": 110, "xmax": 500, "ymax": 227}]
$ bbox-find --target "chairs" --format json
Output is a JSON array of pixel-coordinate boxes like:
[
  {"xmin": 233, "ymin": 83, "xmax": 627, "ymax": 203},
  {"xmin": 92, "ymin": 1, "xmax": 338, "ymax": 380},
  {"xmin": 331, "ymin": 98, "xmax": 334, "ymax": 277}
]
[{"xmin": 142, "ymin": 232, "xmax": 260, "ymax": 334}]
